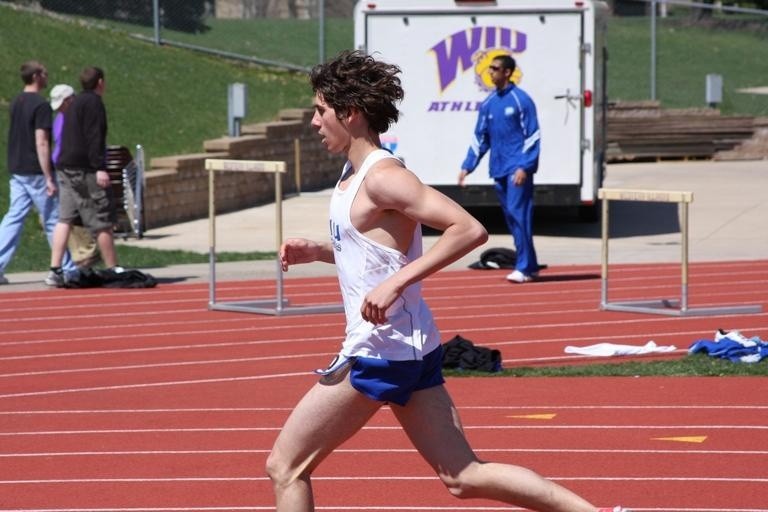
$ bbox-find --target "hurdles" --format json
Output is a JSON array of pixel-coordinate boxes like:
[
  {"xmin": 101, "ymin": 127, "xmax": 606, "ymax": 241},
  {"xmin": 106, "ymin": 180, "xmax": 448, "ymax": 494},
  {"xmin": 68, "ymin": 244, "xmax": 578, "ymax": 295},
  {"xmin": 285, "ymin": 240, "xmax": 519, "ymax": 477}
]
[
  {"xmin": 597, "ymin": 189, "xmax": 762, "ymax": 316},
  {"xmin": 205, "ymin": 160, "xmax": 344, "ymax": 315}
]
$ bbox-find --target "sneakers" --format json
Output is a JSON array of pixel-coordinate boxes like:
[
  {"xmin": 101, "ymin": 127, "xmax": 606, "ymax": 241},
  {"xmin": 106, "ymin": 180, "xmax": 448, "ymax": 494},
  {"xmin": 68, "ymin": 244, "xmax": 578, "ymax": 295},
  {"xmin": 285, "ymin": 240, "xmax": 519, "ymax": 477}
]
[
  {"xmin": 0, "ymin": 266, "xmax": 128, "ymax": 288},
  {"xmin": 507, "ymin": 268, "xmax": 539, "ymax": 283}
]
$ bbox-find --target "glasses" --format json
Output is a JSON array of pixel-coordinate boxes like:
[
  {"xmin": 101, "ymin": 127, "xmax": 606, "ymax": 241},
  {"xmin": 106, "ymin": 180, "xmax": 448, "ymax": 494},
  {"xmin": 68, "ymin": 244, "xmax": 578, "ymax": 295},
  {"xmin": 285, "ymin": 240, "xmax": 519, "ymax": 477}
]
[{"xmin": 489, "ymin": 64, "xmax": 504, "ymax": 71}]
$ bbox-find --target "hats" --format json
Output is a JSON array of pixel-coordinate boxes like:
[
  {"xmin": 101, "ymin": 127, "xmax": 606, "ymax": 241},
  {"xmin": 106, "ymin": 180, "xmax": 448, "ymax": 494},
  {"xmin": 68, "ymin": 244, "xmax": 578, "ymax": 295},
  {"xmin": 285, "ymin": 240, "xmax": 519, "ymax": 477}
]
[{"xmin": 49, "ymin": 83, "xmax": 75, "ymax": 112}]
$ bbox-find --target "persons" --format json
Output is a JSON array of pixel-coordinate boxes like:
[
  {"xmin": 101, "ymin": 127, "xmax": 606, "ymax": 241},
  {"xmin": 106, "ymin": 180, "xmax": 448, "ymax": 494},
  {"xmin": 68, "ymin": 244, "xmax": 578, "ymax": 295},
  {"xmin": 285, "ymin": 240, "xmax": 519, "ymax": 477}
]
[
  {"xmin": 263, "ymin": 47, "xmax": 637, "ymax": 512},
  {"xmin": 0, "ymin": 60, "xmax": 97, "ymax": 289},
  {"xmin": 39, "ymin": 65, "xmax": 120, "ymax": 286},
  {"xmin": 455, "ymin": 54, "xmax": 543, "ymax": 284},
  {"xmin": 46, "ymin": 83, "xmax": 98, "ymax": 269}
]
